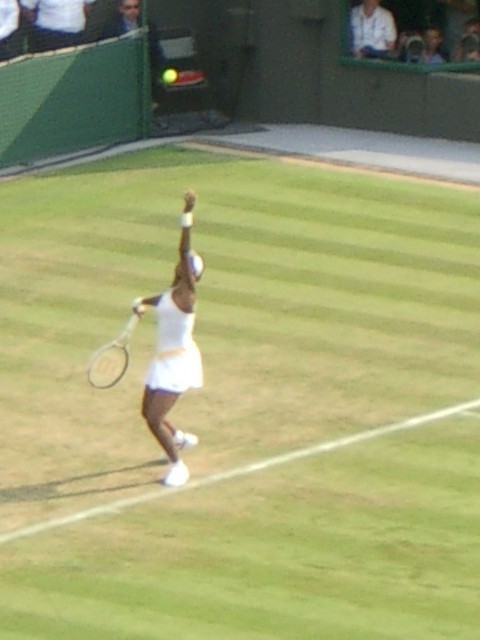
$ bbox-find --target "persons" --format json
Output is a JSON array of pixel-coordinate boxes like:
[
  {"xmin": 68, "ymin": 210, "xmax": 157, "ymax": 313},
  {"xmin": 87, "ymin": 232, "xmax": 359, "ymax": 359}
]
[
  {"xmin": 450, "ymin": 17, "xmax": 480, "ymax": 63},
  {"xmin": 0, "ymin": 0, "xmax": 23, "ymax": 62},
  {"xmin": 350, "ymin": 0, "xmax": 398, "ymax": 59},
  {"xmin": 417, "ymin": 14, "xmax": 447, "ymax": 64},
  {"xmin": 20, "ymin": 0, "xmax": 97, "ymax": 53},
  {"xmin": 101, "ymin": 0, "xmax": 141, "ymax": 40},
  {"xmin": 132, "ymin": 191, "xmax": 205, "ymax": 487}
]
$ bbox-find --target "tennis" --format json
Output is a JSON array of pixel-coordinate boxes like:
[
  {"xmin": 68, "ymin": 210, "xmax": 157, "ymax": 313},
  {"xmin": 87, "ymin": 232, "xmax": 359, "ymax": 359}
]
[{"xmin": 161, "ymin": 68, "xmax": 178, "ymax": 84}]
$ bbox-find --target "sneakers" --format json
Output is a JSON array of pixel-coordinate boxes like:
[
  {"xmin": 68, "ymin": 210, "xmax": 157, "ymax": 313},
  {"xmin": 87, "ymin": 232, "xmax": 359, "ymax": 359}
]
[
  {"xmin": 175, "ymin": 429, "xmax": 198, "ymax": 452},
  {"xmin": 164, "ymin": 460, "xmax": 188, "ymax": 486}
]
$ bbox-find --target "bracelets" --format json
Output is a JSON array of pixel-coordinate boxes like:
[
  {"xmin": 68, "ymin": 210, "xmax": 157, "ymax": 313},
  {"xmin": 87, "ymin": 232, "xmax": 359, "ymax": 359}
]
[
  {"xmin": 179, "ymin": 212, "xmax": 193, "ymax": 228},
  {"xmin": 132, "ymin": 297, "xmax": 142, "ymax": 308}
]
